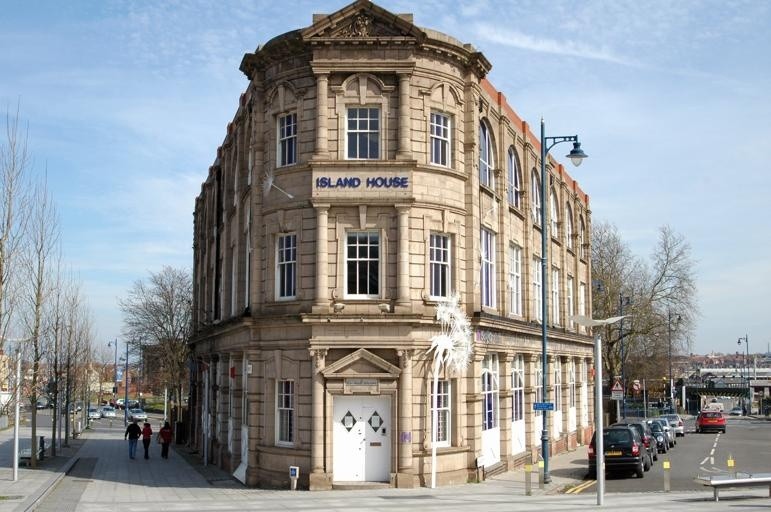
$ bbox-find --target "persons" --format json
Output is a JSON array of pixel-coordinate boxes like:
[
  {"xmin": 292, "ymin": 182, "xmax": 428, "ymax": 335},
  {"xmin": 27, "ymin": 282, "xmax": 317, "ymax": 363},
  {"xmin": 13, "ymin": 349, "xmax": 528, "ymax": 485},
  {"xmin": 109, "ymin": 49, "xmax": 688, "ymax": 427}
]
[
  {"xmin": 124, "ymin": 420, "xmax": 141, "ymax": 459},
  {"xmin": 155, "ymin": 421, "xmax": 173, "ymax": 459},
  {"xmin": 141, "ymin": 423, "xmax": 153, "ymax": 459}
]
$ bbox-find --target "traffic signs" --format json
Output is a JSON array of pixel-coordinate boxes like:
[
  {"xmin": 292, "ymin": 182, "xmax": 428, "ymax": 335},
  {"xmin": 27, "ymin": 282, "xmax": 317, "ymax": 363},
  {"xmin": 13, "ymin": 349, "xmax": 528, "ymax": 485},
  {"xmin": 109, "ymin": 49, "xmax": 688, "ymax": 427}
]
[{"xmin": 533, "ymin": 402, "xmax": 555, "ymax": 410}]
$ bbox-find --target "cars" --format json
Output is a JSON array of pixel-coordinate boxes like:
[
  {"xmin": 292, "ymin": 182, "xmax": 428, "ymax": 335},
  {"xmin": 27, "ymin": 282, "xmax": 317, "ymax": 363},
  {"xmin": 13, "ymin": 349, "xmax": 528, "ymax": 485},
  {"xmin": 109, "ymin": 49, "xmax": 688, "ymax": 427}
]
[
  {"xmin": 587, "ymin": 412, "xmax": 685, "ymax": 480},
  {"xmin": 730, "ymin": 407, "xmax": 743, "ymax": 416},
  {"xmin": 694, "ymin": 410, "xmax": 727, "ymax": 434},
  {"xmin": 703, "ymin": 397, "xmax": 725, "ymax": 412},
  {"xmin": 37, "ymin": 397, "xmax": 149, "ymax": 424}
]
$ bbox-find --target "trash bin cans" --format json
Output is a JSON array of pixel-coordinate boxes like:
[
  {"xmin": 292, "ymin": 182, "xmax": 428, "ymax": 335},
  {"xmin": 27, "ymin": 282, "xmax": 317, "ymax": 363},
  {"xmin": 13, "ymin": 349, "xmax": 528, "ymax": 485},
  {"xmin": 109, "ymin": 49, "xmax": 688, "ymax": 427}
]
[{"xmin": 39, "ymin": 436, "xmax": 45, "ymax": 461}]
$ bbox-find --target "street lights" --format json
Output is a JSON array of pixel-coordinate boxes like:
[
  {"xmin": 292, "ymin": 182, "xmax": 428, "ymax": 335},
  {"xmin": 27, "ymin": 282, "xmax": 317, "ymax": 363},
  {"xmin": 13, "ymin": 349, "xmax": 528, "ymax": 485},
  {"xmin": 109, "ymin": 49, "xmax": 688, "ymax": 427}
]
[
  {"xmin": 733, "ymin": 334, "xmax": 751, "ymax": 418},
  {"xmin": 1, "ymin": 335, "xmax": 36, "ymax": 481},
  {"xmin": 618, "ymin": 291, "xmax": 637, "ymax": 419},
  {"xmin": 667, "ymin": 307, "xmax": 683, "ymax": 398},
  {"xmin": 539, "ymin": 115, "xmax": 589, "ymax": 486},
  {"xmin": 108, "ymin": 338, "xmax": 118, "ymax": 408}
]
